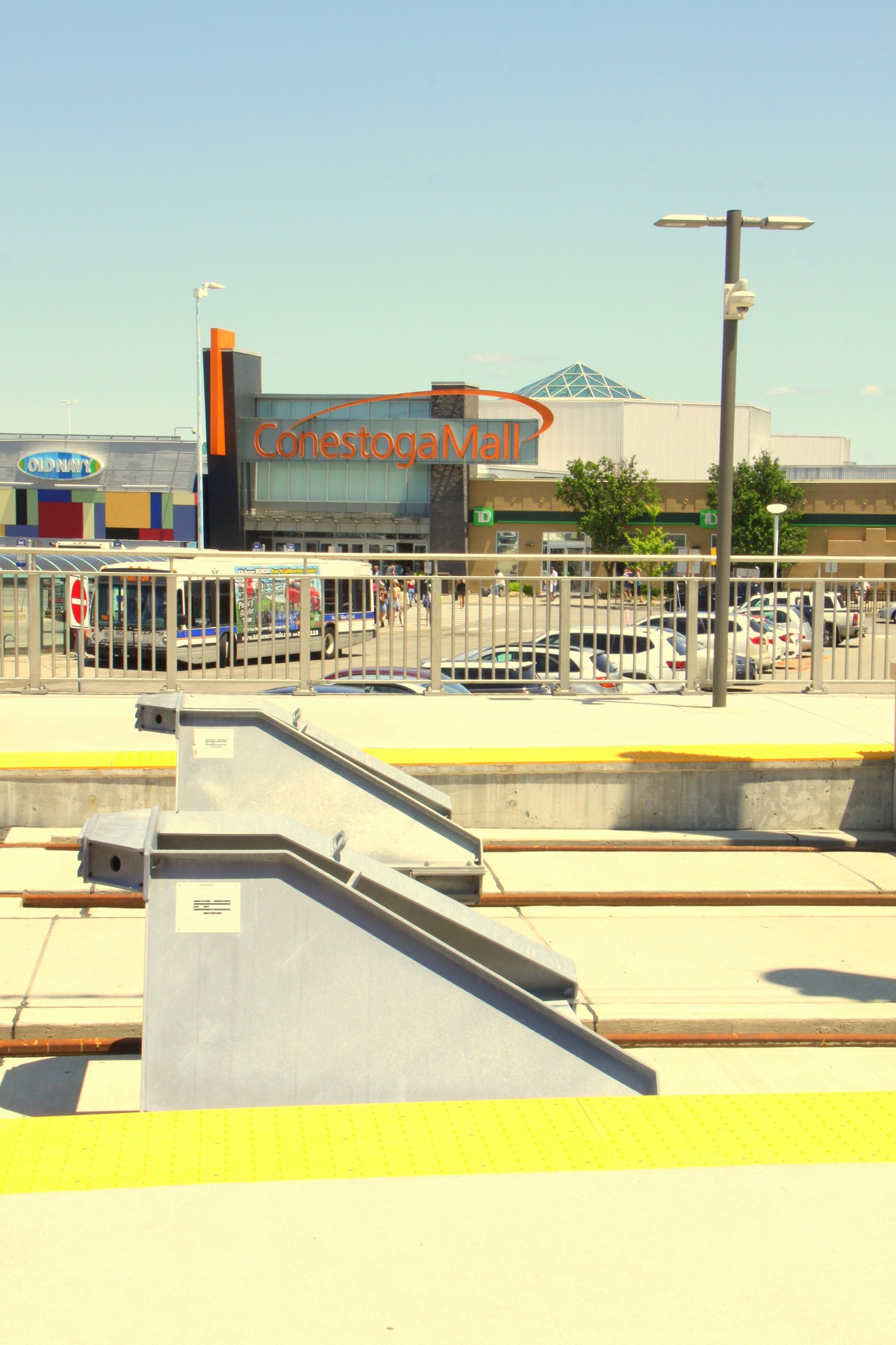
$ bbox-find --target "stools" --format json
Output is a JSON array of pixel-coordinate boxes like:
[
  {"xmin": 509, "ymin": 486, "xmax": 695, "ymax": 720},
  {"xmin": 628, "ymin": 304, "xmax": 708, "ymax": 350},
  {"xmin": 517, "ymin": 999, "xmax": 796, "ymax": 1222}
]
[{"xmin": 499, "ymin": 587, "xmax": 506, "ymax": 597}]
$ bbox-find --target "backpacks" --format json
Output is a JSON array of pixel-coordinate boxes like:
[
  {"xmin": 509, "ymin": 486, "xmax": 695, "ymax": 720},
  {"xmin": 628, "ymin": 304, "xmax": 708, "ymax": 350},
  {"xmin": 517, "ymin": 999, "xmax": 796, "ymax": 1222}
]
[
  {"xmin": 424, "ymin": 593, "xmax": 430, "ymax": 608},
  {"xmin": 379, "ymin": 593, "xmax": 385, "ymax": 604}
]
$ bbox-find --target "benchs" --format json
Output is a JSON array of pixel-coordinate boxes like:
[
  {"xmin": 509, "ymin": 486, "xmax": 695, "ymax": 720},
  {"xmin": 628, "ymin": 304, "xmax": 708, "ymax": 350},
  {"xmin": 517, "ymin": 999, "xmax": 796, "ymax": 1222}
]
[{"xmin": 613, "ymin": 587, "xmax": 631, "ymax": 603}]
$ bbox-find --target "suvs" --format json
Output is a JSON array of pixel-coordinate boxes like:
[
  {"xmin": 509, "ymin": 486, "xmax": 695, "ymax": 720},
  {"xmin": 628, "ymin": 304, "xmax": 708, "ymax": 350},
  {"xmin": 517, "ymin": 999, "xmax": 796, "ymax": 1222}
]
[
  {"xmin": 420, "ymin": 660, "xmax": 552, "ymax": 695},
  {"xmin": 532, "ymin": 624, "xmax": 687, "ymax": 693},
  {"xmin": 663, "ymin": 580, "xmax": 768, "ymax": 612},
  {"xmin": 468, "ymin": 641, "xmax": 623, "ymax": 695}
]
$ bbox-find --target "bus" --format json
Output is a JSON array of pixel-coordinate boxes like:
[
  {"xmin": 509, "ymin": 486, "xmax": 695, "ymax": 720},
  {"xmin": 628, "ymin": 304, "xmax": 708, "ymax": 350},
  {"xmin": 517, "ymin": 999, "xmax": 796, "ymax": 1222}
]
[{"xmin": 86, "ymin": 556, "xmax": 375, "ymax": 670}]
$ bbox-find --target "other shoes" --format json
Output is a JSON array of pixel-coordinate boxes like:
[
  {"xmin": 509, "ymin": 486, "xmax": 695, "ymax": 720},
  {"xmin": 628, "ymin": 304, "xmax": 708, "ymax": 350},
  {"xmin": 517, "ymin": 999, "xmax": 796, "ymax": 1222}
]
[{"xmin": 380, "ymin": 622, "xmax": 384, "ymax": 627}]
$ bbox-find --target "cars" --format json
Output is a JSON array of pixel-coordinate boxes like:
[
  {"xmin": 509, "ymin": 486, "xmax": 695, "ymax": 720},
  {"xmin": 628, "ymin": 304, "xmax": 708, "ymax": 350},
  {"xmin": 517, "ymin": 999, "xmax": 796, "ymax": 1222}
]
[
  {"xmin": 878, "ymin": 601, "xmax": 896, "ymax": 624},
  {"xmin": 695, "ymin": 640, "xmax": 756, "ymax": 680},
  {"xmin": 255, "ymin": 684, "xmax": 382, "ymax": 694},
  {"xmin": 322, "ymin": 666, "xmax": 473, "ymax": 694},
  {"xmin": 628, "ymin": 590, "xmax": 868, "ymax": 673}
]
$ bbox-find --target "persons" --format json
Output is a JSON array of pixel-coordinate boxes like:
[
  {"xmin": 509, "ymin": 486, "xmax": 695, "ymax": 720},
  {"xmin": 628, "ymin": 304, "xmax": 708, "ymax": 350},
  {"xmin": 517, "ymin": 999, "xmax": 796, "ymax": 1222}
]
[
  {"xmin": 487, "ymin": 569, "xmax": 505, "ymax": 598},
  {"xmin": 373, "ymin": 565, "xmax": 380, "ymax": 576},
  {"xmin": 628, "ymin": 566, "xmax": 644, "ymax": 603},
  {"xmin": 543, "ymin": 580, "xmax": 547, "ymax": 602},
  {"xmin": 549, "ymin": 566, "xmax": 558, "ymax": 601},
  {"xmin": 407, "ymin": 579, "xmax": 417, "ymax": 607},
  {"xmin": 510, "ymin": 565, "xmax": 517, "ymax": 582},
  {"xmin": 371, "ymin": 579, "xmax": 410, "ymax": 628},
  {"xmin": 854, "ymin": 576, "xmax": 870, "ymax": 604},
  {"xmin": 623, "ymin": 569, "xmax": 633, "ymax": 584},
  {"xmin": 394, "ymin": 561, "xmax": 404, "ymax": 583},
  {"xmin": 385, "ymin": 561, "xmax": 397, "ymax": 579},
  {"xmin": 622, "ymin": 567, "xmax": 635, "ymax": 602},
  {"xmin": 421, "ymin": 586, "xmax": 431, "ymax": 621},
  {"xmin": 456, "ymin": 580, "xmax": 465, "ymax": 608}
]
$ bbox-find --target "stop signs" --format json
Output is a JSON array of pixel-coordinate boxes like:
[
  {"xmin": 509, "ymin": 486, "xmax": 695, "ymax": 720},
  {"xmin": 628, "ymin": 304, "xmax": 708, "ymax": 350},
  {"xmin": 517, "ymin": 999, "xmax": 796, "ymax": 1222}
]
[{"xmin": 66, "ymin": 575, "xmax": 90, "ymax": 629}]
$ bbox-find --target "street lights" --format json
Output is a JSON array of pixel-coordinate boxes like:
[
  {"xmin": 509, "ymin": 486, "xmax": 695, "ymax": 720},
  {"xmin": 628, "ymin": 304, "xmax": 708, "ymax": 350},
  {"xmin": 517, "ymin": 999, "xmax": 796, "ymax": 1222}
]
[
  {"xmin": 653, "ymin": 209, "xmax": 816, "ymax": 707},
  {"xmin": 61, "ymin": 400, "xmax": 79, "ymax": 438},
  {"xmin": 767, "ymin": 504, "xmax": 788, "ymax": 677},
  {"xmin": 191, "ymin": 282, "xmax": 225, "ymax": 550}
]
